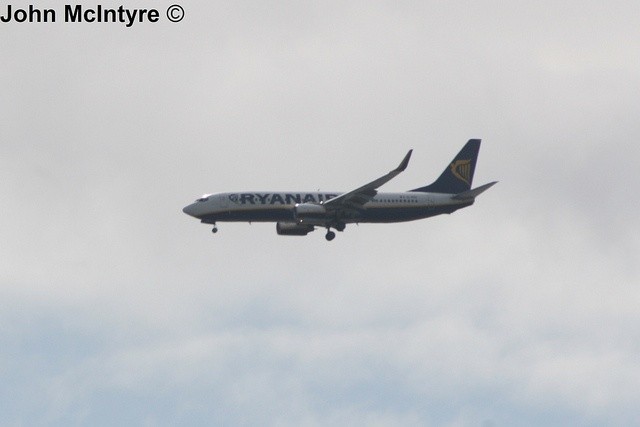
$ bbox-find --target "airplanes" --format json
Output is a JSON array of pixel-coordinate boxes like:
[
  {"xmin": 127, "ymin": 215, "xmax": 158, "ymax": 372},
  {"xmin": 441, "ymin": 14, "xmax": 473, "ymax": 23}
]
[{"xmin": 183, "ymin": 139, "xmax": 498, "ymax": 240}]
